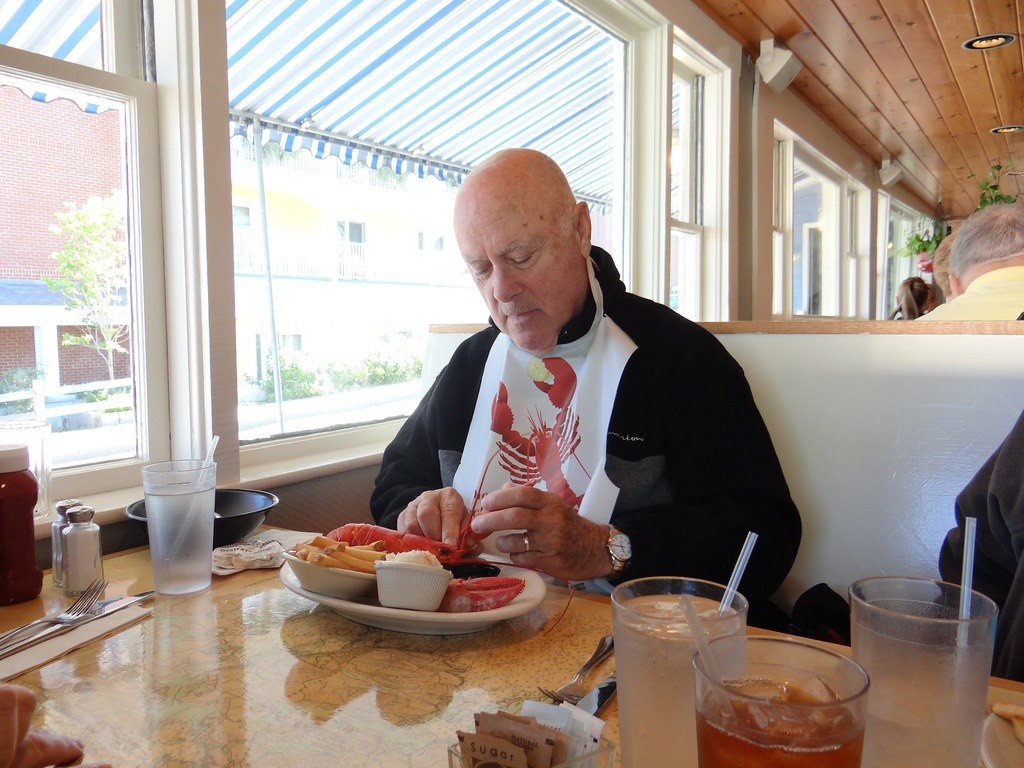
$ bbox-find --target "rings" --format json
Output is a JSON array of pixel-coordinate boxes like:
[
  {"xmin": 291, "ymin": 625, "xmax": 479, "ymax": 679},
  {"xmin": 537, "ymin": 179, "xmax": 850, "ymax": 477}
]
[{"xmin": 524, "ymin": 532, "xmax": 530, "ymax": 552}]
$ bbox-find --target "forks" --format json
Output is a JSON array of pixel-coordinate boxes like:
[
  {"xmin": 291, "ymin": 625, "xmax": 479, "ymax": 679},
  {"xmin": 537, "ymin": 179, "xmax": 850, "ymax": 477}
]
[
  {"xmin": 0, "ymin": 579, "xmax": 109, "ymax": 646},
  {"xmin": 537, "ymin": 635, "xmax": 615, "ymax": 704}
]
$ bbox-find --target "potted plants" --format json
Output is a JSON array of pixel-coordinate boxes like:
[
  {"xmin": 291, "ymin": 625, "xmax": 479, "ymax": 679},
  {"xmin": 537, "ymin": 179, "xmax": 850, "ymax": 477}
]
[{"xmin": 884, "ymin": 220, "xmax": 950, "ymax": 270}]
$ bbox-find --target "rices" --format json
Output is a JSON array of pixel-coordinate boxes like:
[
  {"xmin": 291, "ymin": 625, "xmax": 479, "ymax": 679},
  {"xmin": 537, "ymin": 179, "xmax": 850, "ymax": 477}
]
[{"xmin": 385, "ymin": 550, "xmax": 443, "ymax": 569}]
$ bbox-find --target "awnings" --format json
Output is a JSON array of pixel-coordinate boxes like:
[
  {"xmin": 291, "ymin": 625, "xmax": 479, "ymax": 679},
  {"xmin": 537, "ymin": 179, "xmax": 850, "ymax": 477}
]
[{"xmin": 0, "ymin": 0, "xmax": 809, "ymax": 221}]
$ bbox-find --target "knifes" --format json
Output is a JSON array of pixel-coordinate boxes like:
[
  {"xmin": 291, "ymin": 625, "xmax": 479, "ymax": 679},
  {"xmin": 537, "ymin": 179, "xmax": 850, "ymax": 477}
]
[
  {"xmin": 0, "ymin": 591, "xmax": 155, "ymax": 660},
  {"xmin": 574, "ymin": 673, "xmax": 617, "ymax": 715}
]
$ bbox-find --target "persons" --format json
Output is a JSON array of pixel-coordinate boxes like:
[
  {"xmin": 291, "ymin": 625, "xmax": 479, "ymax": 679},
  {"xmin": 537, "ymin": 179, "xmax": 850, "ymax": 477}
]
[
  {"xmin": 914, "ymin": 202, "xmax": 1024, "ymax": 320},
  {"xmin": 921, "ymin": 409, "xmax": 1024, "ymax": 682},
  {"xmin": 887, "ymin": 276, "xmax": 946, "ymax": 320},
  {"xmin": 932, "ymin": 230, "xmax": 958, "ymax": 303},
  {"xmin": 0, "ymin": 684, "xmax": 112, "ymax": 768},
  {"xmin": 370, "ymin": 148, "xmax": 801, "ymax": 618}
]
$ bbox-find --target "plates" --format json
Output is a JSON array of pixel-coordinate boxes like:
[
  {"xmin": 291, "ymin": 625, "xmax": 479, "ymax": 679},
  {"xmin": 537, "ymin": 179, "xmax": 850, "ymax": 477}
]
[
  {"xmin": 279, "ymin": 561, "xmax": 545, "ymax": 635},
  {"xmin": 981, "ymin": 710, "xmax": 1024, "ymax": 768}
]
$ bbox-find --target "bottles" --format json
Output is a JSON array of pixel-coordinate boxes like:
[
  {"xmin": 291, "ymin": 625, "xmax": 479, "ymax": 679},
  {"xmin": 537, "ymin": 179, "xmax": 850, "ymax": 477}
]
[
  {"xmin": 52, "ymin": 498, "xmax": 104, "ymax": 596},
  {"xmin": 0, "ymin": 445, "xmax": 43, "ymax": 605}
]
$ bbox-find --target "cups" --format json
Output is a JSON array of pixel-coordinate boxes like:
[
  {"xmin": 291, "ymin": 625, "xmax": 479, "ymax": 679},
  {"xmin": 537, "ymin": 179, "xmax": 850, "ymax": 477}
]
[
  {"xmin": 848, "ymin": 577, "xmax": 999, "ymax": 768},
  {"xmin": 692, "ymin": 635, "xmax": 871, "ymax": 768},
  {"xmin": 611, "ymin": 577, "xmax": 748, "ymax": 768},
  {"xmin": 141, "ymin": 459, "xmax": 217, "ymax": 594},
  {"xmin": 0, "ymin": 420, "xmax": 51, "ymax": 520}
]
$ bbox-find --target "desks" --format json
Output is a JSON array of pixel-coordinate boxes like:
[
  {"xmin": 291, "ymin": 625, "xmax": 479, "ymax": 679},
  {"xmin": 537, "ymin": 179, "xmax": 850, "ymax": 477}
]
[{"xmin": 0, "ymin": 525, "xmax": 1024, "ymax": 768}]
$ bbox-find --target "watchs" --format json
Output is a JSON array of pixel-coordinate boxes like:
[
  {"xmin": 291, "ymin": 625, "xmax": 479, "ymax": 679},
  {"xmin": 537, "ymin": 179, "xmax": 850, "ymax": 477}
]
[{"xmin": 605, "ymin": 524, "xmax": 632, "ymax": 581}]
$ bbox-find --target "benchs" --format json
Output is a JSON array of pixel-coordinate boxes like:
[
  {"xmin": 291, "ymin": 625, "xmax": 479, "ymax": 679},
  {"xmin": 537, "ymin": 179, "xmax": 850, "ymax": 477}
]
[{"xmin": 685, "ymin": 321, "xmax": 1024, "ymax": 612}]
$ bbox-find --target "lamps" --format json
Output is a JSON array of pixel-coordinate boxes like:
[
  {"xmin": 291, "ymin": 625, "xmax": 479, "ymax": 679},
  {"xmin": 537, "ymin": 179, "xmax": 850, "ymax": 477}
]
[
  {"xmin": 878, "ymin": 149, "xmax": 904, "ymax": 191},
  {"xmin": 752, "ymin": 36, "xmax": 805, "ymax": 94}
]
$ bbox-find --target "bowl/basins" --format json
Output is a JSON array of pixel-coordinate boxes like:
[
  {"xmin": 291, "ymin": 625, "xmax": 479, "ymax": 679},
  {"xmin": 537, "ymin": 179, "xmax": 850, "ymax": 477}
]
[
  {"xmin": 373, "ymin": 561, "xmax": 453, "ymax": 611},
  {"xmin": 125, "ymin": 489, "xmax": 279, "ymax": 552},
  {"xmin": 281, "ymin": 550, "xmax": 377, "ymax": 603}
]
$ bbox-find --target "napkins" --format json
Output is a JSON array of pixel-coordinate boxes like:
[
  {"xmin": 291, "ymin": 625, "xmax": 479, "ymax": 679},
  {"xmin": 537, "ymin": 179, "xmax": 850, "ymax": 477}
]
[{"xmin": 0, "ymin": 595, "xmax": 152, "ymax": 683}]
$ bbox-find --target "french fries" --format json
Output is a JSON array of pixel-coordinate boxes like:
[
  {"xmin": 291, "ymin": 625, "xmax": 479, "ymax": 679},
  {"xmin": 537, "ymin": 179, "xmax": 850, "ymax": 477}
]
[{"xmin": 294, "ymin": 535, "xmax": 387, "ymax": 573}]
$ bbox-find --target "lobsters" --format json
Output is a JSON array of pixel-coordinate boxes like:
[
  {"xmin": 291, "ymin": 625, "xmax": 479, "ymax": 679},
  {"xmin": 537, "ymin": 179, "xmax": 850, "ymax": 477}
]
[{"xmin": 323, "ymin": 432, "xmax": 577, "ymax": 636}]
